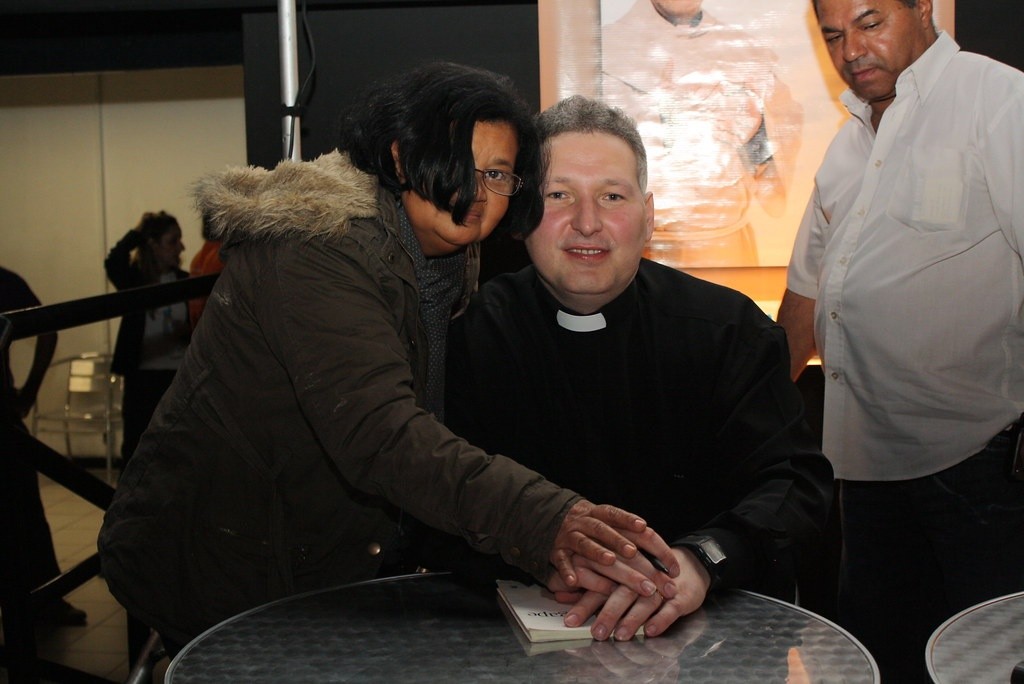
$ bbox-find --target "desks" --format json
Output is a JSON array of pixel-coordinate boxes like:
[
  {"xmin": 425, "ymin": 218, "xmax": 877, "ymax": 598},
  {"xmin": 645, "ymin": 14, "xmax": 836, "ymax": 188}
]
[
  {"xmin": 924, "ymin": 590, "xmax": 1024, "ymax": 684},
  {"xmin": 162, "ymin": 571, "xmax": 880, "ymax": 683}
]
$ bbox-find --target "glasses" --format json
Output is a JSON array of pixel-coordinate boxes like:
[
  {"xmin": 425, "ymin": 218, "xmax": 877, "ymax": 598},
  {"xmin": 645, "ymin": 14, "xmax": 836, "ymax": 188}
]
[{"xmin": 473, "ymin": 167, "xmax": 523, "ymax": 197}]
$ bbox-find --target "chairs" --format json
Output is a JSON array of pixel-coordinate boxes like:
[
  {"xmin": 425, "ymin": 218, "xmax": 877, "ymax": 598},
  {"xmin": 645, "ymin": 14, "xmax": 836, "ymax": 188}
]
[{"xmin": 25, "ymin": 352, "xmax": 125, "ymax": 489}]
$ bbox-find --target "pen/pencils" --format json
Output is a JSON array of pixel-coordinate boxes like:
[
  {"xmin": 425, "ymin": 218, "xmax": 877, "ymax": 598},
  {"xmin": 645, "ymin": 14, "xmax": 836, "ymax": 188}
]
[{"xmin": 638, "ymin": 548, "xmax": 671, "ymax": 576}]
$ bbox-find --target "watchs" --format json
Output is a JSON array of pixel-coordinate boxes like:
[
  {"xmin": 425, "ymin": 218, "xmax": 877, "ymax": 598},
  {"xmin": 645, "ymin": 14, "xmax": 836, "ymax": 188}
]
[{"xmin": 669, "ymin": 535, "xmax": 727, "ymax": 591}]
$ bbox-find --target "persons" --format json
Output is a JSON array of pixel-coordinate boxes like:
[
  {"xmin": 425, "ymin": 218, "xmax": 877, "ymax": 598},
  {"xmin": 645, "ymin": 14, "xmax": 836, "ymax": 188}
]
[
  {"xmin": 0, "ymin": 266, "xmax": 88, "ymax": 623},
  {"xmin": 447, "ymin": 101, "xmax": 835, "ymax": 641},
  {"xmin": 774, "ymin": 0, "xmax": 1024, "ymax": 684},
  {"xmin": 97, "ymin": 61, "xmax": 647, "ymax": 662},
  {"xmin": 104, "ymin": 210, "xmax": 190, "ymax": 484},
  {"xmin": 598, "ymin": 0, "xmax": 780, "ymax": 263},
  {"xmin": 556, "ymin": 595, "xmax": 812, "ymax": 684},
  {"xmin": 188, "ymin": 214, "xmax": 225, "ymax": 330}
]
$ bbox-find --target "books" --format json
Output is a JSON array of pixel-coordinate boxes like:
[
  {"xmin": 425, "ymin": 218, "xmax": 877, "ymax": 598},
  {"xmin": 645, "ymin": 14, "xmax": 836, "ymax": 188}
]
[
  {"xmin": 497, "ymin": 593, "xmax": 644, "ymax": 656},
  {"xmin": 496, "ymin": 579, "xmax": 644, "ymax": 643}
]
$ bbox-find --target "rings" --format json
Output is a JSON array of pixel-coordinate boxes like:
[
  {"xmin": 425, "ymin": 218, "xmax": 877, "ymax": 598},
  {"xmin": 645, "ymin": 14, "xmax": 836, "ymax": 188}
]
[{"xmin": 656, "ymin": 589, "xmax": 663, "ymax": 597}]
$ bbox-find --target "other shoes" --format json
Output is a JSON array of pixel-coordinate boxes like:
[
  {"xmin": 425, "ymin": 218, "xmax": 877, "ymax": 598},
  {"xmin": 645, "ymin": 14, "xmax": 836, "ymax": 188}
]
[{"xmin": 47, "ymin": 599, "xmax": 86, "ymax": 622}]
